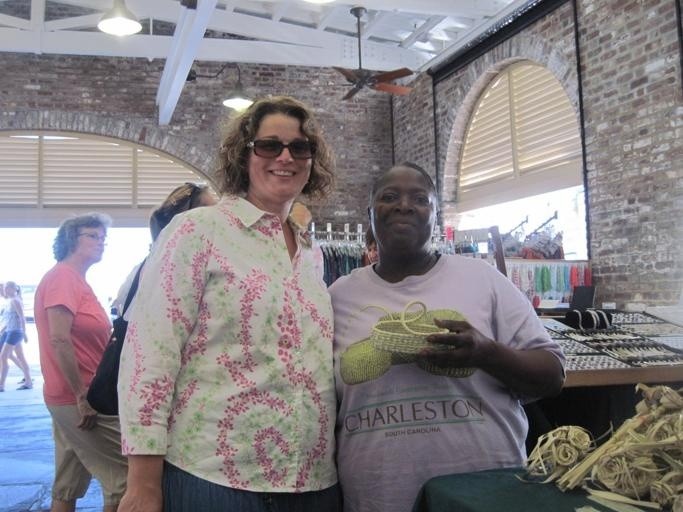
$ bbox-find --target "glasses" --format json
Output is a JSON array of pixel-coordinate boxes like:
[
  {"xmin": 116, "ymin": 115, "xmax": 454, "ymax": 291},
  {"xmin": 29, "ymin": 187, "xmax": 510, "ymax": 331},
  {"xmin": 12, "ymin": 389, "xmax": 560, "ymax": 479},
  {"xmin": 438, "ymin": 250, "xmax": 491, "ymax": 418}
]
[
  {"xmin": 77, "ymin": 231, "xmax": 109, "ymax": 247},
  {"xmin": 243, "ymin": 137, "xmax": 313, "ymax": 161}
]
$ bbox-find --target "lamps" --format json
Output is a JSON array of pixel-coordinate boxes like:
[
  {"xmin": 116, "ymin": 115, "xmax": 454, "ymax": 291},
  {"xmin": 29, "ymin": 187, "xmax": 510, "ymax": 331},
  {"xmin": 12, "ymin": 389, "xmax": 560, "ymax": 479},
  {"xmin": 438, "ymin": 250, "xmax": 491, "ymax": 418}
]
[
  {"xmin": 93, "ymin": 2, "xmax": 140, "ymax": 40},
  {"xmin": 188, "ymin": 57, "xmax": 253, "ymax": 114}
]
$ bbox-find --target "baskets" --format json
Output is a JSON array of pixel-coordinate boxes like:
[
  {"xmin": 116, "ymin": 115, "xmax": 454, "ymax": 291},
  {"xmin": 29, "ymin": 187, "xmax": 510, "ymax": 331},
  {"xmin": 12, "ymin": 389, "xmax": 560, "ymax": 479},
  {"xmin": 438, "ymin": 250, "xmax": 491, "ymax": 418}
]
[{"xmin": 371, "ymin": 302, "xmax": 448, "ymax": 356}]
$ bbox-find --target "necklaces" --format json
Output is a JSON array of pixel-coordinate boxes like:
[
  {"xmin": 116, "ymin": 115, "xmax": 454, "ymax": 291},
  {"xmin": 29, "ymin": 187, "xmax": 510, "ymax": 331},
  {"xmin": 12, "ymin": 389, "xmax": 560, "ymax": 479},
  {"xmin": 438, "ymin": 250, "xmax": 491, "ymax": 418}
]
[{"xmin": 574, "ymin": 310, "xmax": 610, "ymax": 331}]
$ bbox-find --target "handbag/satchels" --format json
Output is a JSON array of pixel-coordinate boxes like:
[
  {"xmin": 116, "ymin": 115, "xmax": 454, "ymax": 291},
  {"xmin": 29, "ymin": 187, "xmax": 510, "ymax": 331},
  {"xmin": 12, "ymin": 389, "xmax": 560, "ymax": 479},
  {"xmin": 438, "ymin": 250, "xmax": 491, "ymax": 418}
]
[{"xmin": 84, "ymin": 316, "xmax": 127, "ymax": 415}]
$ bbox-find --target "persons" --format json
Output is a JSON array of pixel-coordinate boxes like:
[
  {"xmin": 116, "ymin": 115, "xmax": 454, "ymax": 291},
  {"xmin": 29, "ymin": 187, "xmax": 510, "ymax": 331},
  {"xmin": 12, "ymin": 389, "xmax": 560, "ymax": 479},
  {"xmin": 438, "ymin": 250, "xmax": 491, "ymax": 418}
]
[
  {"xmin": 33, "ymin": 216, "xmax": 129, "ymax": 512},
  {"xmin": 117, "ymin": 98, "xmax": 341, "ymax": 512},
  {"xmin": 328, "ymin": 163, "xmax": 565, "ymax": 512},
  {"xmin": 365, "ymin": 225, "xmax": 378, "ymax": 264},
  {"xmin": 116, "ymin": 184, "xmax": 217, "ymax": 322}
]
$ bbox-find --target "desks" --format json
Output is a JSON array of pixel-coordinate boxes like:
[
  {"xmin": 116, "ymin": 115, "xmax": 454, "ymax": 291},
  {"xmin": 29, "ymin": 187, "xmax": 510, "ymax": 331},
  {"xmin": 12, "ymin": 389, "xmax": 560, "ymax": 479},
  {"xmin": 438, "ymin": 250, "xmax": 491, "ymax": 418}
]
[{"xmin": 412, "ymin": 451, "xmax": 681, "ymax": 511}]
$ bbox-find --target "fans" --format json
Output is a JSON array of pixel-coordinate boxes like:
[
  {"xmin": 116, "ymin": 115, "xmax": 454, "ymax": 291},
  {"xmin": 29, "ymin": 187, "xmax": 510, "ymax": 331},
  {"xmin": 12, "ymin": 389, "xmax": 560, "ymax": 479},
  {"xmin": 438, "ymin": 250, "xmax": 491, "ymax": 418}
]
[{"xmin": 330, "ymin": 8, "xmax": 413, "ymax": 102}]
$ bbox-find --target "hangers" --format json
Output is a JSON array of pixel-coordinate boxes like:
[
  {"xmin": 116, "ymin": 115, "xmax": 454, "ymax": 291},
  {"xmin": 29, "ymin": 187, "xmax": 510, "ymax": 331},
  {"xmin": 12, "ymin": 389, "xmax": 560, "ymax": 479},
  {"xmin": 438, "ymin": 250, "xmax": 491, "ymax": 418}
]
[
  {"xmin": 303, "ymin": 229, "xmax": 453, "ymax": 254},
  {"xmin": 503, "ymin": 220, "xmax": 561, "ymax": 257}
]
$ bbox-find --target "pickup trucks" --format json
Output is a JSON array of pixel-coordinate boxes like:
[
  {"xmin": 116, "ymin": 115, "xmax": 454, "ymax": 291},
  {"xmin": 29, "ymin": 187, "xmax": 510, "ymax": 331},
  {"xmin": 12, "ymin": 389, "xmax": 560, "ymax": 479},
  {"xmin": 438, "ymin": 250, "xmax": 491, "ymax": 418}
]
[{"xmin": 17, "ymin": 284, "xmax": 39, "ymax": 321}]
